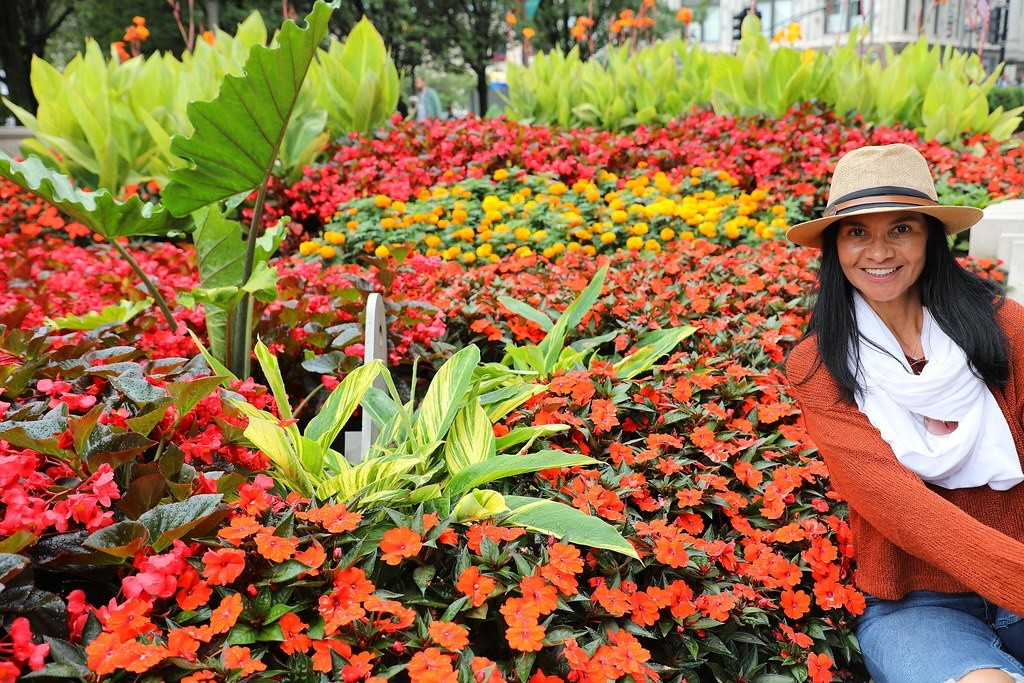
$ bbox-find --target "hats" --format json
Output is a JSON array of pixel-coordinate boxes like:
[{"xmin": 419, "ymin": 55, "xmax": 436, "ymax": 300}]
[{"xmin": 786, "ymin": 143, "xmax": 983, "ymax": 248}]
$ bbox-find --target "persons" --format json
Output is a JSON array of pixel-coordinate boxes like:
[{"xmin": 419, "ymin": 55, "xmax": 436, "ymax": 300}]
[
  {"xmin": 685, "ymin": 22, "xmax": 701, "ymax": 52},
  {"xmin": 398, "ymin": 93, "xmax": 409, "ymax": 119},
  {"xmin": 410, "ymin": 76, "xmax": 441, "ymax": 121},
  {"xmin": 785, "ymin": 146, "xmax": 1024, "ymax": 683}
]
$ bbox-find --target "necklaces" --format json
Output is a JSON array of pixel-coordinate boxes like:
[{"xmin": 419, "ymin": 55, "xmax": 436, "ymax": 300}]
[{"xmin": 892, "ymin": 329, "xmax": 921, "ymax": 360}]
